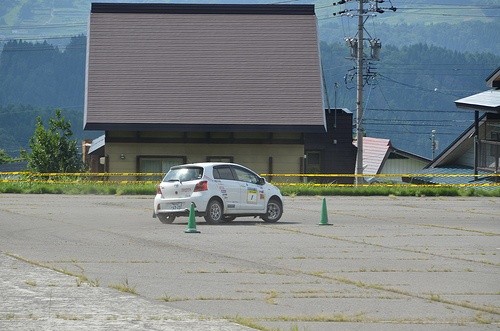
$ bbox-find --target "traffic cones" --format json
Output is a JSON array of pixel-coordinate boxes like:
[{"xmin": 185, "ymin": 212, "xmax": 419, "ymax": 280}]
[
  {"xmin": 184, "ymin": 203, "xmax": 201, "ymax": 233},
  {"xmin": 317, "ymin": 197, "xmax": 334, "ymax": 226}
]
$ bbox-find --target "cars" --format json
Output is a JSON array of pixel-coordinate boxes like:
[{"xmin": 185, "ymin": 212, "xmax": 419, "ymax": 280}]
[{"xmin": 151, "ymin": 161, "xmax": 285, "ymax": 225}]
